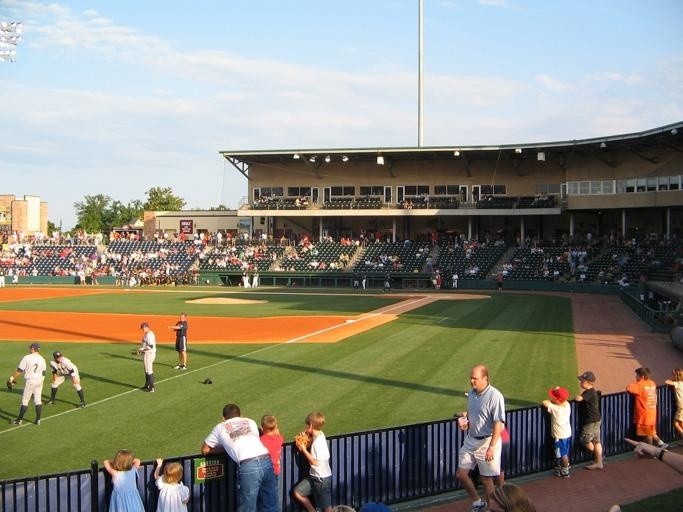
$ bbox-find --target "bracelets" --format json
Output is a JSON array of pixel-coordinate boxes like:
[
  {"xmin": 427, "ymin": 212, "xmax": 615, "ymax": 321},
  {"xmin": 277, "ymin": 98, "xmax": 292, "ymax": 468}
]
[{"xmin": 658, "ymin": 449, "xmax": 668, "ymax": 461}]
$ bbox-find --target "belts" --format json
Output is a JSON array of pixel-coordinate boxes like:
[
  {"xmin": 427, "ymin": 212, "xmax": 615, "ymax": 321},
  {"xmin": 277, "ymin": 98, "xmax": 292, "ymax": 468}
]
[{"xmin": 474, "ymin": 436, "xmax": 489, "ymax": 440}]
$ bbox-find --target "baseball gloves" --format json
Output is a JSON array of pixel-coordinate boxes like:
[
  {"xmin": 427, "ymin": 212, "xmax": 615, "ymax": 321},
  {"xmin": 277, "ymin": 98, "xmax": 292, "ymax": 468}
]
[
  {"xmin": 7, "ymin": 379, "xmax": 15, "ymax": 390},
  {"xmin": 130, "ymin": 349, "xmax": 142, "ymax": 357}
]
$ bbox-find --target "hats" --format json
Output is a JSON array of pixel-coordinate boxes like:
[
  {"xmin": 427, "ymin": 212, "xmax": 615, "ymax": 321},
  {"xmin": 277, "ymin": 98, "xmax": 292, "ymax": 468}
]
[
  {"xmin": 577, "ymin": 372, "xmax": 595, "ymax": 382},
  {"xmin": 360, "ymin": 502, "xmax": 393, "ymax": 512},
  {"xmin": 31, "ymin": 343, "xmax": 40, "ymax": 349},
  {"xmin": 549, "ymin": 386, "xmax": 569, "ymax": 401},
  {"xmin": 53, "ymin": 351, "xmax": 62, "ymax": 357}
]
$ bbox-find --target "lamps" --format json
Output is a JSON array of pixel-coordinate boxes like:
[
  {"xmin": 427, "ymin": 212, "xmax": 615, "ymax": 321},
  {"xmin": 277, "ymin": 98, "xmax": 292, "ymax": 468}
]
[
  {"xmin": 293, "ymin": 154, "xmax": 349, "ymax": 169},
  {"xmin": 377, "ymin": 152, "xmax": 385, "ymax": 167},
  {"xmin": 599, "ymin": 143, "xmax": 608, "ymax": 148},
  {"xmin": 537, "ymin": 149, "xmax": 546, "ymax": 161},
  {"xmin": 671, "ymin": 130, "xmax": 676, "ymax": 134}
]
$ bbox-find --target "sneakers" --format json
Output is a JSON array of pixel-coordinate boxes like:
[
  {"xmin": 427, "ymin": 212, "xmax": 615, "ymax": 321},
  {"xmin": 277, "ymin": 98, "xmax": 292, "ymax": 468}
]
[
  {"xmin": 141, "ymin": 385, "xmax": 154, "ymax": 392},
  {"xmin": 658, "ymin": 443, "xmax": 669, "ymax": 449},
  {"xmin": 10, "ymin": 419, "xmax": 41, "ymax": 425},
  {"xmin": 47, "ymin": 400, "xmax": 54, "ymax": 405},
  {"xmin": 584, "ymin": 463, "xmax": 604, "ymax": 469},
  {"xmin": 470, "ymin": 497, "xmax": 487, "ymax": 512},
  {"xmin": 554, "ymin": 462, "xmax": 572, "ymax": 477},
  {"xmin": 80, "ymin": 402, "xmax": 87, "ymax": 408},
  {"xmin": 174, "ymin": 364, "xmax": 187, "ymax": 370}
]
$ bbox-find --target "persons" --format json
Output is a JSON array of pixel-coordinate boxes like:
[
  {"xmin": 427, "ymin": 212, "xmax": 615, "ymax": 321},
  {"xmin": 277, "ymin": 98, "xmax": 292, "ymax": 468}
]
[
  {"xmin": 455, "ymin": 365, "xmax": 506, "ymax": 512},
  {"xmin": 168, "ymin": 313, "xmax": 188, "ymax": 370},
  {"xmin": 7, "ymin": 343, "xmax": 46, "ymax": 425},
  {"xmin": 624, "ymin": 437, "xmax": 683, "ymax": 475},
  {"xmin": 353, "ymin": 228, "xmax": 504, "ymax": 292},
  {"xmin": 0, "ymin": 228, "xmax": 191, "ymax": 287},
  {"xmin": 486, "ymin": 483, "xmax": 537, "ymax": 512},
  {"xmin": 294, "ymin": 412, "xmax": 332, "ymax": 512},
  {"xmin": 103, "ymin": 449, "xmax": 146, "ymax": 512},
  {"xmin": 658, "ymin": 298, "xmax": 681, "ymax": 340},
  {"xmin": 260, "ymin": 415, "xmax": 283, "ymax": 478},
  {"xmin": 495, "ymin": 229, "xmax": 682, "ymax": 303},
  {"xmin": 137, "ymin": 323, "xmax": 157, "ymax": 392},
  {"xmin": 664, "ymin": 368, "xmax": 683, "ymax": 437},
  {"xmin": 626, "ymin": 367, "xmax": 669, "ymax": 458},
  {"xmin": 542, "ymin": 386, "xmax": 572, "ymax": 477},
  {"xmin": 325, "ymin": 505, "xmax": 357, "ymax": 512},
  {"xmin": 201, "ymin": 403, "xmax": 279, "ymax": 512},
  {"xmin": 185, "ymin": 229, "xmax": 374, "ymax": 288},
  {"xmin": 253, "ymin": 188, "xmax": 548, "ymax": 206},
  {"xmin": 574, "ymin": 371, "xmax": 604, "ymax": 469},
  {"xmin": 154, "ymin": 458, "xmax": 189, "ymax": 512},
  {"xmin": 47, "ymin": 351, "xmax": 85, "ymax": 408}
]
